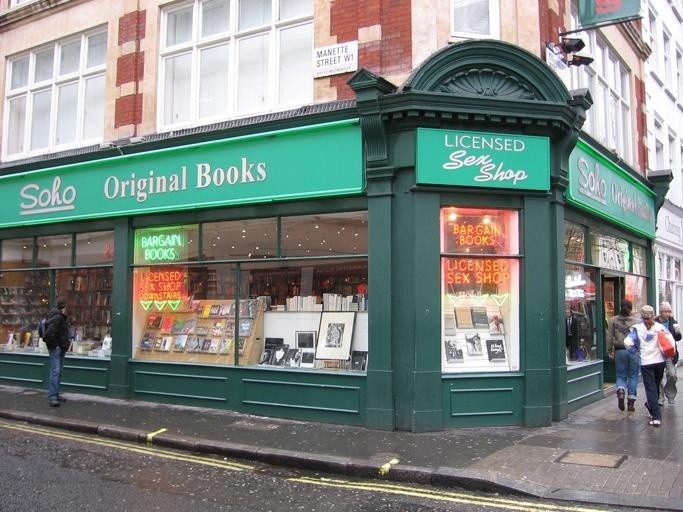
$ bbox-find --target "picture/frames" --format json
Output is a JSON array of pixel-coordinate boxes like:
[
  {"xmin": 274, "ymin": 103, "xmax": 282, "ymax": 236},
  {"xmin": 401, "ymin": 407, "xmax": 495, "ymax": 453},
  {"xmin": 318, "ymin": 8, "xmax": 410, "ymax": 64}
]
[
  {"xmin": 313, "ymin": 310, "xmax": 356, "ymax": 361},
  {"xmin": 293, "ymin": 330, "xmax": 317, "ymax": 369}
]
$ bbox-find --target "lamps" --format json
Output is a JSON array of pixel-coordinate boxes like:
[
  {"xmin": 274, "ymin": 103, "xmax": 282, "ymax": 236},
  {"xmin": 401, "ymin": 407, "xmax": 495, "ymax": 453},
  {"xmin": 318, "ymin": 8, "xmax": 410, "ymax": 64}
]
[{"xmin": 547, "ymin": 36, "xmax": 592, "ymax": 69}]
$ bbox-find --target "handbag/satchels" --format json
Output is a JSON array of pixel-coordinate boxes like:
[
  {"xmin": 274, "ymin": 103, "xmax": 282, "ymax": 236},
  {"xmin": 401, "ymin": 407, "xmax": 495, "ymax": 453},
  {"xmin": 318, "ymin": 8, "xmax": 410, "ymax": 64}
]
[
  {"xmin": 39, "ymin": 317, "xmax": 47, "ymax": 339},
  {"xmin": 657, "ymin": 330, "xmax": 675, "ymax": 358}
]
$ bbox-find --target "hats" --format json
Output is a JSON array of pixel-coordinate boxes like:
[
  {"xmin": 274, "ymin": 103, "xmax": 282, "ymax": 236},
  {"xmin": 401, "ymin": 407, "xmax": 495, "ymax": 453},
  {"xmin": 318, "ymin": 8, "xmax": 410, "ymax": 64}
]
[{"xmin": 660, "ymin": 301, "xmax": 672, "ymax": 312}]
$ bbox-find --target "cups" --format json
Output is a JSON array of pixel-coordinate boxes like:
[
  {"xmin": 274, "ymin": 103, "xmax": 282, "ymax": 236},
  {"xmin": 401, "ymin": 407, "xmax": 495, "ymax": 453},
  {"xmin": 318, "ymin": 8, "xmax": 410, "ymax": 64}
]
[{"xmin": 673, "ymin": 324, "xmax": 679, "ymax": 332}]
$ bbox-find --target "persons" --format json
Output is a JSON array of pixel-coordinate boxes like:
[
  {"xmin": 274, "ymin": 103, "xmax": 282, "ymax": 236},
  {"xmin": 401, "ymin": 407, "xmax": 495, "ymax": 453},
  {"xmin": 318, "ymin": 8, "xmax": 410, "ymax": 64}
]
[
  {"xmin": 565, "ymin": 307, "xmax": 595, "ymax": 360},
  {"xmin": 42, "ymin": 294, "xmax": 74, "ymax": 408},
  {"xmin": 606, "ymin": 299, "xmax": 643, "ymax": 409},
  {"xmin": 654, "ymin": 302, "xmax": 683, "ymax": 405},
  {"xmin": 622, "ymin": 305, "xmax": 672, "ymax": 426}
]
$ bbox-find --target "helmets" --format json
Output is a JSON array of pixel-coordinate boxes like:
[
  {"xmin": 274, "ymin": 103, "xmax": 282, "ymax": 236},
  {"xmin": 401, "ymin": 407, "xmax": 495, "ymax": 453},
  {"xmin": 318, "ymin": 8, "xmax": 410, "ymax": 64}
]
[{"xmin": 641, "ymin": 305, "xmax": 654, "ymax": 314}]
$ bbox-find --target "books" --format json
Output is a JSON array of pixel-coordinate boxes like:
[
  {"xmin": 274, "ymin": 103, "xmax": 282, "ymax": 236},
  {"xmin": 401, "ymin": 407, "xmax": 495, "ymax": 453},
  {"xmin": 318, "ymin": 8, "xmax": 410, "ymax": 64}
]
[
  {"xmin": 70, "ymin": 277, "xmax": 112, "ymax": 341},
  {"xmin": 140, "ymin": 300, "xmax": 258, "ymax": 354},
  {"xmin": 188, "ymin": 275, "xmax": 299, "ymax": 307},
  {"xmin": 444, "ymin": 339, "xmax": 463, "ymax": 362},
  {"xmin": 454, "ymin": 307, "xmax": 473, "ymax": 328},
  {"xmin": 258, "ymin": 293, "xmax": 369, "ymax": 371},
  {"xmin": 443, "ymin": 311, "xmax": 457, "ymax": 335},
  {"xmin": 486, "ymin": 311, "xmax": 505, "ymax": 334},
  {"xmin": 465, "ymin": 333, "xmax": 482, "ymax": 355},
  {"xmin": 1, "ymin": 287, "xmax": 112, "ymax": 358},
  {"xmin": 471, "ymin": 306, "xmax": 488, "ymax": 327},
  {"xmin": 485, "ymin": 339, "xmax": 504, "ymax": 361}
]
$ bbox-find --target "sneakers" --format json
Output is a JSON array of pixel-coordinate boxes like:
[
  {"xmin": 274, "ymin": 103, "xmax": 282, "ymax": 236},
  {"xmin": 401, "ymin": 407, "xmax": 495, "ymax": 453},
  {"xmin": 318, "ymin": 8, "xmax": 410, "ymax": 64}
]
[
  {"xmin": 50, "ymin": 400, "xmax": 60, "ymax": 407},
  {"xmin": 59, "ymin": 396, "xmax": 65, "ymax": 401}
]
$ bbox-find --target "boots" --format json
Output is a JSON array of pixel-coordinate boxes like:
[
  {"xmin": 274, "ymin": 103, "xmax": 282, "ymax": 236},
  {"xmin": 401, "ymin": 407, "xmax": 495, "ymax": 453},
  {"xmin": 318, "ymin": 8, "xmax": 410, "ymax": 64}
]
[
  {"xmin": 627, "ymin": 399, "xmax": 635, "ymax": 411},
  {"xmin": 617, "ymin": 388, "xmax": 624, "ymax": 410}
]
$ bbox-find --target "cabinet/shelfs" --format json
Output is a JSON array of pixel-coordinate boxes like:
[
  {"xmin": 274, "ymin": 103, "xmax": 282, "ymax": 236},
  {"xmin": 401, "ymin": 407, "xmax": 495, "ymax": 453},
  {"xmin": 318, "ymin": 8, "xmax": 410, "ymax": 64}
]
[
  {"xmin": 0, "ymin": 283, "xmax": 47, "ymax": 328},
  {"xmin": 181, "ymin": 261, "xmax": 368, "ymax": 306},
  {"xmin": 132, "ymin": 299, "xmax": 264, "ymax": 366},
  {"xmin": 56, "ymin": 266, "xmax": 110, "ymax": 343}
]
